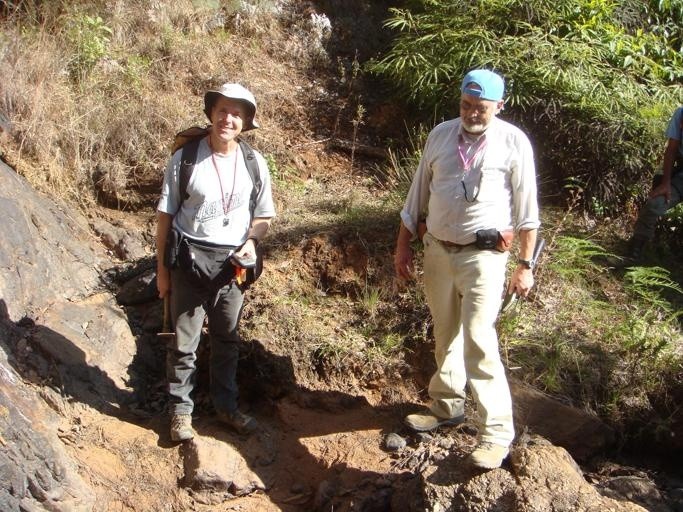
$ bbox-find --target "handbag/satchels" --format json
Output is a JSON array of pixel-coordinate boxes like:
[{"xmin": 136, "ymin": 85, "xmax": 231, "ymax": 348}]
[{"xmin": 476, "ymin": 229, "xmax": 513, "ymax": 251}]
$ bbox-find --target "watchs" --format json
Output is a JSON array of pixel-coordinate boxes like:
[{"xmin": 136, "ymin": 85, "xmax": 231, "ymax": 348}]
[{"xmin": 517, "ymin": 257, "xmax": 536, "ymax": 269}]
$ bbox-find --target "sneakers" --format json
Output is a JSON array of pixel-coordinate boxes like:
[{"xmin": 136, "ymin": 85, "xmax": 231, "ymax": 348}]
[
  {"xmin": 403, "ymin": 410, "xmax": 466, "ymax": 431},
  {"xmin": 468, "ymin": 441, "xmax": 509, "ymax": 469},
  {"xmin": 216, "ymin": 409, "xmax": 256, "ymax": 432},
  {"xmin": 170, "ymin": 414, "xmax": 192, "ymax": 441}
]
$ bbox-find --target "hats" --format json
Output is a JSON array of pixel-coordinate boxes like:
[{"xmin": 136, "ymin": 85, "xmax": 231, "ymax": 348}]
[
  {"xmin": 461, "ymin": 69, "xmax": 505, "ymax": 101},
  {"xmin": 203, "ymin": 83, "xmax": 259, "ymax": 131}
]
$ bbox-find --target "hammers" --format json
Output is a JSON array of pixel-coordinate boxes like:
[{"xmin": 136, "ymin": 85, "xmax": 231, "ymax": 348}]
[{"xmin": 157, "ymin": 293, "xmax": 176, "ymax": 337}]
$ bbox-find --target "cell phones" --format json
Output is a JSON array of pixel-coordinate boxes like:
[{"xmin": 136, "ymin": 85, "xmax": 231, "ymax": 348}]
[{"xmin": 233, "ymin": 252, "xmax": 256, "ymax": 268}]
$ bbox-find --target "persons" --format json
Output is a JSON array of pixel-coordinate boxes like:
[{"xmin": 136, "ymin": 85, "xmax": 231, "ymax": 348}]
[
  {"xmin": 392, "ymin": 68, "xmax": 539, "ymax": 468},
  {"xmin": 155, "ymin": 83, "xmax": 276, "ymax": 440},
  {"xmin": 614, "ymin": 104, "xmax": 683, "ymax": 281}
]
